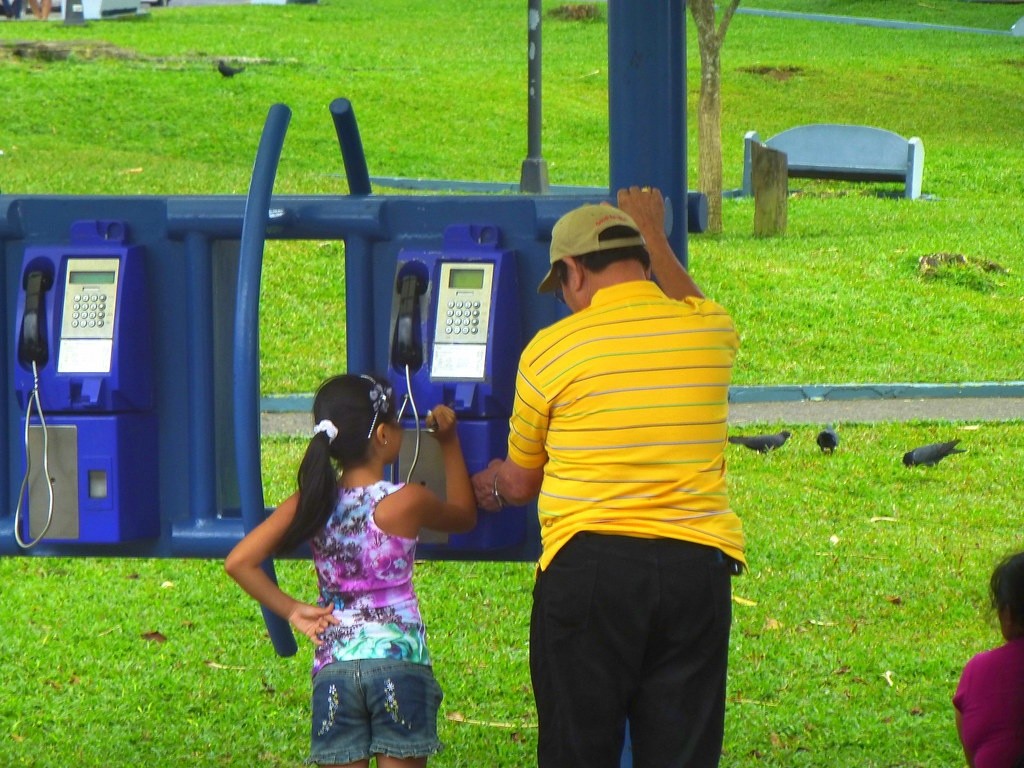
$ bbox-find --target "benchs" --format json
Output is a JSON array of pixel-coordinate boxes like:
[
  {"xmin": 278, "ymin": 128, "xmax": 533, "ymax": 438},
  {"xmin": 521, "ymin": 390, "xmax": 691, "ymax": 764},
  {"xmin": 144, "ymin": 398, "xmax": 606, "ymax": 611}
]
[{"xmin": 744, "ymin": 124, "xmax": 925, "ymax": 200}]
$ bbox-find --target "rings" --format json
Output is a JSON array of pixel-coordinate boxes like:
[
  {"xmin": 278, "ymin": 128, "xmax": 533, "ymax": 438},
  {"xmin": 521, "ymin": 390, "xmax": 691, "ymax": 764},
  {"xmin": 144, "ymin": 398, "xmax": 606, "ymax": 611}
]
[{"xmin": 642, "ymin": 187, "xmax": 650, "ymax": 192}]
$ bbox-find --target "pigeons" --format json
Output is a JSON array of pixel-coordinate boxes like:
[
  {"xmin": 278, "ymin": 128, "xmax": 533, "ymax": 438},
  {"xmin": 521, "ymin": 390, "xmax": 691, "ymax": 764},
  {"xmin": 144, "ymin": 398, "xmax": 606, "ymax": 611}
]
[
  {"xmin": 217, "ymin": 58, "xmax": 246, "ymax": 80},
  {"xmin": 815, "ymin": 427, "xmax": 840, "ymax": 457},
  {"xmin": 727, "ymin": 429, "xmax": 792, "ymax": 456},
  {"xmin": 902, "ymin": 438, "xmax": 968, "ymax": 469}
]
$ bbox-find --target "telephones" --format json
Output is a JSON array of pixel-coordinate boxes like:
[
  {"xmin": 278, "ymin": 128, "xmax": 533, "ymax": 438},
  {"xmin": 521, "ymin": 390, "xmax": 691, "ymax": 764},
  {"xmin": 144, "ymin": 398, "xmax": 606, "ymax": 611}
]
[
  {"xmin": 14, "ymin": 242, "xmax": 154, "ymax": 415},
  {"xmin": 386, "ymin": 243, "xmax": 519, "ymax": 420}
]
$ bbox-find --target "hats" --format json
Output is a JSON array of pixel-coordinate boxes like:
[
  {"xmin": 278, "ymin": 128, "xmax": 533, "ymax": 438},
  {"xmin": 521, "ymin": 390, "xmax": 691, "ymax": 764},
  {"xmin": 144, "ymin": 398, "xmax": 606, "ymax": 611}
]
[{"xmin": 537, "ymin": 203, "xmax": 646, "ymax": 294}]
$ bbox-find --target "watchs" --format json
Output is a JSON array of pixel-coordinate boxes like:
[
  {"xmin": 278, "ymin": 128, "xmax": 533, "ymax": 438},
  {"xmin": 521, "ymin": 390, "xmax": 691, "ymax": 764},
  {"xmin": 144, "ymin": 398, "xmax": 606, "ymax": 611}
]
[{"xmin": 492, "ymin": 472, "xmax": 506, "ymax": 507}]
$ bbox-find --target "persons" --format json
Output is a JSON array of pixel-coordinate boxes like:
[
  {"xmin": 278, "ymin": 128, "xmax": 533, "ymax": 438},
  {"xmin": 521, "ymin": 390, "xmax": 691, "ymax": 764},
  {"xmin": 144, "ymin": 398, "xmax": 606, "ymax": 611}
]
[
  {"xmin": 950, "ymin": 553, "xmax": 1024, "ymax": 768},
  {"xmin": 226, "ymin": 372, "xmax": 479, "ymax": 767},
  {"xmin": 472, "ymin": 186, "xmax": 749, "ymax": 767},
  {"xmin": 29, "ymin": 0, "xmax": 52, "ymax": 22},
  {"xmin": 3, "ymin": 0, "xmax": 22, "ymax": 20}
]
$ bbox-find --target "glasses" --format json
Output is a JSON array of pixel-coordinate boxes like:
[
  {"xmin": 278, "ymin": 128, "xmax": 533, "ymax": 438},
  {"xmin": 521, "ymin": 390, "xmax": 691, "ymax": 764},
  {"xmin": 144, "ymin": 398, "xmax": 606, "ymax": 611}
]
[{"xmin": 553, "ymin": 272, "xmax": 568, "ymax": 305}]
